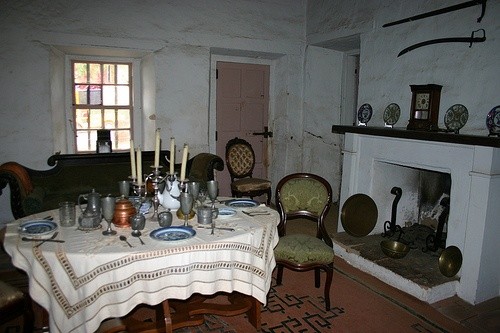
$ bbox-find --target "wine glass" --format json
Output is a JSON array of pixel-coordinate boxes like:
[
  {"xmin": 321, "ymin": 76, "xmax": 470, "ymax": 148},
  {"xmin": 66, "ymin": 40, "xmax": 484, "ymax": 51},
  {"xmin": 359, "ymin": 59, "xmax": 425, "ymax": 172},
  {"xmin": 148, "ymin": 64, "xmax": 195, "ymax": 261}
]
[
  {"xmin": 101, "ymin": 197, "xmax": 117, "ymax": 236},
  {"xmin": 181, "ymin": 181, "xmax": 218, "ymax": 226}
]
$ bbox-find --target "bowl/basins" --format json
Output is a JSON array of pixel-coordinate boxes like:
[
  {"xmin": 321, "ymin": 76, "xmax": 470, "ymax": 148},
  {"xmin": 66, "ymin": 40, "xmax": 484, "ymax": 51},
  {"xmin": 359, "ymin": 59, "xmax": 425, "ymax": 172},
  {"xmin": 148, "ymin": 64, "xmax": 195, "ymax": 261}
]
[{"xmin": 380, "ymin": 240, "xmax": 409, "ymax": 259}]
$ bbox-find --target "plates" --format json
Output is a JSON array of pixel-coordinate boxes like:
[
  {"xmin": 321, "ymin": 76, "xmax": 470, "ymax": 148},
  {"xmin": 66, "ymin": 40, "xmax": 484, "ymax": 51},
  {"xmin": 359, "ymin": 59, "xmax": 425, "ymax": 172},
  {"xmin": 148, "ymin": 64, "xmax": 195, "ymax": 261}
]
[
  {"xmin": 358, "ymin": 103, "xmax": 373, "ymax": 124},
  {"xmin": 224, "ymin": 199, "xmax": 259, "ymax": 209},
  {"xmin": 341, "ymin": 194, "xmax": 379, "ymax": 238},
  {"xmin": 444, "ymin": 104, "xmax": 469, "ymax": 131},
  {"xmin": 18, "ymin": 219, "xmax": 58, "ymax": 236},
  {"xmin": 382, "ymin": 103, "xmax": 401, "ymax": 126},
  {"xmin": 149, "ymin": 226, "xmax": 196, "ymax": 242},
  {"xmin": 485, "ymin": 105, "xmax": 500, "ymax": 134},
  {"xmin": 216, "ymin": 208, "xmax": 237, "ymax": 219},
  {"xmin": 439, "ymin": 246, "xmax": 462, "ymax": 277}
]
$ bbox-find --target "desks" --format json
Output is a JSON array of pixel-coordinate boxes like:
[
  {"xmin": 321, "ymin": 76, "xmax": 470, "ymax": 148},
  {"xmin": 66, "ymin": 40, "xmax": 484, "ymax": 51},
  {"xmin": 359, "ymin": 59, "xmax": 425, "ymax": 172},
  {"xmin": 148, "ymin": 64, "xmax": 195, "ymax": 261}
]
[{"xmin": 0, "ymin": 197, "xmax": 280, "ymax": 333}]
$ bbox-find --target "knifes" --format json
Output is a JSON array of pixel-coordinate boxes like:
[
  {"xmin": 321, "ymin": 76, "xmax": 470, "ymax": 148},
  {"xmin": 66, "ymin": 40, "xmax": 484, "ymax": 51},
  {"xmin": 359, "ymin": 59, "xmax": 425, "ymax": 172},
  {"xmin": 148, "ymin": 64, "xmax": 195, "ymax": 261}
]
[
  {"xmin": 21, "ymin": 237, "xmax": 65, "ymax": 243},
  {"xmin": 197, "ymin": 226, "xmax": 235, "ymax": 232}
]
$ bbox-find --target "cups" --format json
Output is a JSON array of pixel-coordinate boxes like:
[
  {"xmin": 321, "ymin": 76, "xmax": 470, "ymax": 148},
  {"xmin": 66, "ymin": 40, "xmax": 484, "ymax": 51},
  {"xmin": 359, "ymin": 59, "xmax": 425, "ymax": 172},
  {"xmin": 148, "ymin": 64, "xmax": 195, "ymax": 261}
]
[
  {"xmin": 197, "ymin": 205, "xmax": 219, "ymax": 225},
  {"xmin": 118, "ymin": 181, "xmax": 130, "ymax": 196},
  {"xmin": 131, "ymin": 213, "xmax": 146, "ymax": 230},
  {"xmin": 58, "ymin": 201, "xmax": 76, "ymax": 227},
  {"xmin": 158, "ymin": 212, "xmax": 172, "ymax": 228}
]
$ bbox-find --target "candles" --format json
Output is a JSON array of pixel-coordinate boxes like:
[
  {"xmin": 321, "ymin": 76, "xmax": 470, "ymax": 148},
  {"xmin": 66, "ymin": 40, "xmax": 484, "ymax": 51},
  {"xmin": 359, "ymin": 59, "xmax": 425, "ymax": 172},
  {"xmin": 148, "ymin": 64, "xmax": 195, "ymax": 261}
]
[
  {"xmin": 154, "ymin": 128, "xmax": 160, "ymax": 167},
  {"xmin": 130, "ymin": 140, "xmax": 136, "ymax": 177},
  {"xmin": 170, "ymin": 137, "xmax": 175, "ymax": 175},
  {"xmin": 137, "ymin": 145, "xmax": 142, "ymax": 185},
  {"xmin": 180, "ymin": 143, "xmax": 188, "ymax": 180}
]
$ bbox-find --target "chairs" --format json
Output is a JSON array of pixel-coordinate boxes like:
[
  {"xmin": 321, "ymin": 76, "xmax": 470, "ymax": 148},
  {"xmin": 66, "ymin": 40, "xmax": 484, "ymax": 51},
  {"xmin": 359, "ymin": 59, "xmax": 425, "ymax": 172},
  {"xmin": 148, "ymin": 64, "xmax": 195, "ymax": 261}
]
[
  {"xmin": 0, "ymin": 280, "xmax": 34, "ymax": 333},
  {"xmin": 275, "ymin": 173, "xmax": 335, "ymax": 311},
  {"xmin": 225, "ymin": 137, "xmax": 272, "ymax": 205}
]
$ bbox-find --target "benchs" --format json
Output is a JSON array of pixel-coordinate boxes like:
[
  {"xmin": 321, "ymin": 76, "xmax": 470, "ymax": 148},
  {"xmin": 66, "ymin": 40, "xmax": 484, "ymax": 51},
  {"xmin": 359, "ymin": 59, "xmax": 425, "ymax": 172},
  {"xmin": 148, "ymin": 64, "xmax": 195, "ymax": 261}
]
[{"xmin": 0, "ymin": 150, "xmax": 224, "ymax": 220}]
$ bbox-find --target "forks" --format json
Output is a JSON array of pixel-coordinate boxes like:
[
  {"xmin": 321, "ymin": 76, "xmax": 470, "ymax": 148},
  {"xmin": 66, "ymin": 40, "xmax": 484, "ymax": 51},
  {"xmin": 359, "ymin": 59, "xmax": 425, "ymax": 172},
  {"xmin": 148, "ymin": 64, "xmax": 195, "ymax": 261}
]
[
  {"xmin": 210, "ymin": 220, "xmax": 215, "ymax": 236},
  {"xmin": 33, "ymin": 232, "xmax": 59, "ymax": 247}
]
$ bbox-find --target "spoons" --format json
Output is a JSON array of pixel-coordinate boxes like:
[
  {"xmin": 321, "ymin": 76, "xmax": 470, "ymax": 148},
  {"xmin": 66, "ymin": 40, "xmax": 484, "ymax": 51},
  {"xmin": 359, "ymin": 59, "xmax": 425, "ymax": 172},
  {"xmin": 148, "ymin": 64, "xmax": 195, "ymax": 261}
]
[
  {"xmin": 242, "ymin": 210, "xmax": 271, "ymax": 216},
  {"xmin": 131, "ymin": 230, "xmax": 146, "ymax": 245},
  {"xmin": 119, "ymin": 235, "xmax": 132, "ymax": 247}
]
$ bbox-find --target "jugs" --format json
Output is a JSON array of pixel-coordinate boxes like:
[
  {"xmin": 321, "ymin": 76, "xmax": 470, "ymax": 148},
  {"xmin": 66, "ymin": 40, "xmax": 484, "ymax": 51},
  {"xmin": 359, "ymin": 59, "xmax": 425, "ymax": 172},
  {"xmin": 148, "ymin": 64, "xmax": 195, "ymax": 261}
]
[{"xmin": 78, "ymin": 188, "xmax": 103, "ymax": 228}]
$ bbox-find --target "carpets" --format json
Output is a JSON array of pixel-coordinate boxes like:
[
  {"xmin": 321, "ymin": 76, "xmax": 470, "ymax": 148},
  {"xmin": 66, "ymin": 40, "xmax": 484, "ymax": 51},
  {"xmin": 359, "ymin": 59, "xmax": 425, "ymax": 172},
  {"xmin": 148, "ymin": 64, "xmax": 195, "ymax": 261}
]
[{"xmin": 41, "ymin": 250, "xmax": 472, "ymax": 333}]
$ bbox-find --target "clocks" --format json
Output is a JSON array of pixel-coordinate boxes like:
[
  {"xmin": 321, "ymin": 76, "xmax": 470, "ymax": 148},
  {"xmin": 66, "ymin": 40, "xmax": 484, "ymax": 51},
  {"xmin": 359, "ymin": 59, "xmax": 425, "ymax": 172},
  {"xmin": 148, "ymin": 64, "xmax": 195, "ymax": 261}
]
[{"xmin": 407, "ymin": 84, "xmax": 443, "ymax": 132}]
